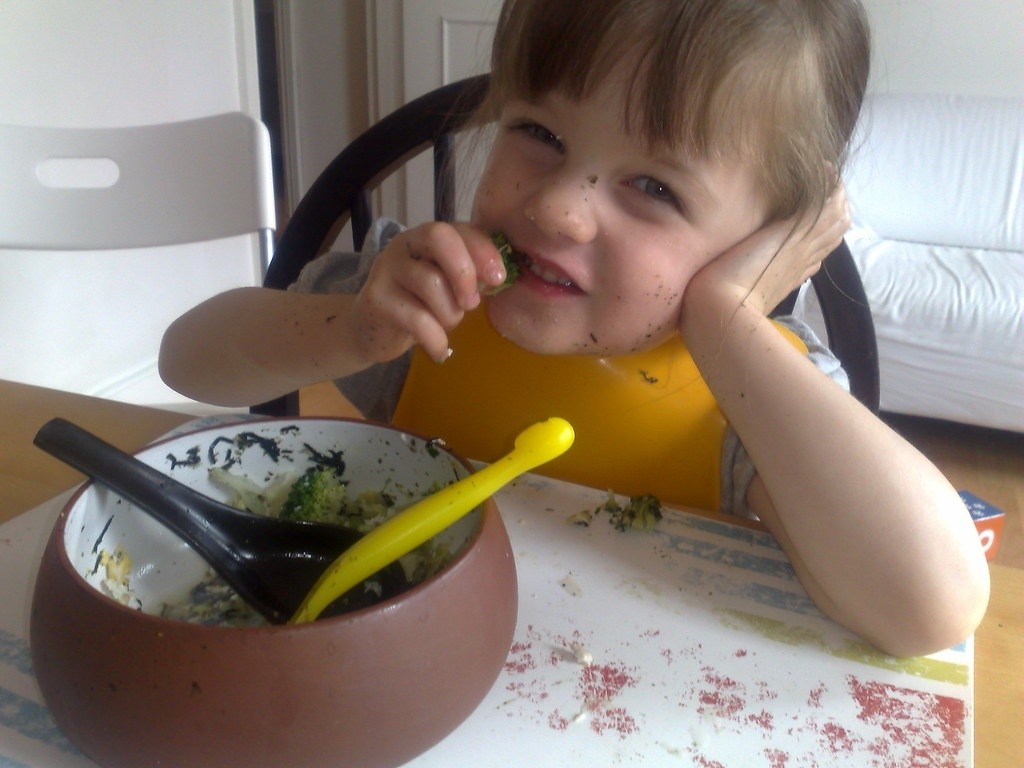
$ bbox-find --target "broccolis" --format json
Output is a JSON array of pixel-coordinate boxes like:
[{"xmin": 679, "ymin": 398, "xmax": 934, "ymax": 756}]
[
  {"xmin": 476, "ymin": 229, "xmax": 517, "ymax": 292},
  {"xmin": 606, "ymin": 491, "xmax": 663, "ymax": 530},
  {"xmin": 280, "ymin": 471, "xmax": 346, "ymax": 524}
]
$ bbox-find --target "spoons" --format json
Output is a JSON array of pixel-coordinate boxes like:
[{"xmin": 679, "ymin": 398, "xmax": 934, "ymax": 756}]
[{"xmin": 32, "ymin": 418, "xmax": 409, "ymax": 623}]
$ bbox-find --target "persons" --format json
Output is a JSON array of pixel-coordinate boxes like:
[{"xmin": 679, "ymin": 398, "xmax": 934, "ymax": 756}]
[{"xmin": 157, "ymin": 0, "xmax": 994, "ymax": 656}]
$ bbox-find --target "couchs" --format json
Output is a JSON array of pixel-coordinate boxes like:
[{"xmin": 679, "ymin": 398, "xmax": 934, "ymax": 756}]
[{"xmin": 791, "ymin": 93, "xmax": 1024, "ymax": 432}]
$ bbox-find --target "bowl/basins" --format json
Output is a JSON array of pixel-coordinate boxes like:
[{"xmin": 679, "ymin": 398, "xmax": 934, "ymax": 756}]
[{"xmin": 30, "ymin": 419, "xmax": 518, "ymax": 768}]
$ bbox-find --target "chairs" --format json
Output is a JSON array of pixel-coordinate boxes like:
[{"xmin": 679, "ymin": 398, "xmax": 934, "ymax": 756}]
[
  {"xmin": 250, "ymin": 73, "xmax": 882, "ymax": 422},
  {"xmin": 0, "ymin": 112, "xmax": 275, "ymax": 416}
]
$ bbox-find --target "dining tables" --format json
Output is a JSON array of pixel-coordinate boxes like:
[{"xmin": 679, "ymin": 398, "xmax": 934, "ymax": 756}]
[{"xmin": 0, "ymin": 376, "xmax": 1024, "ymax": 767}]
